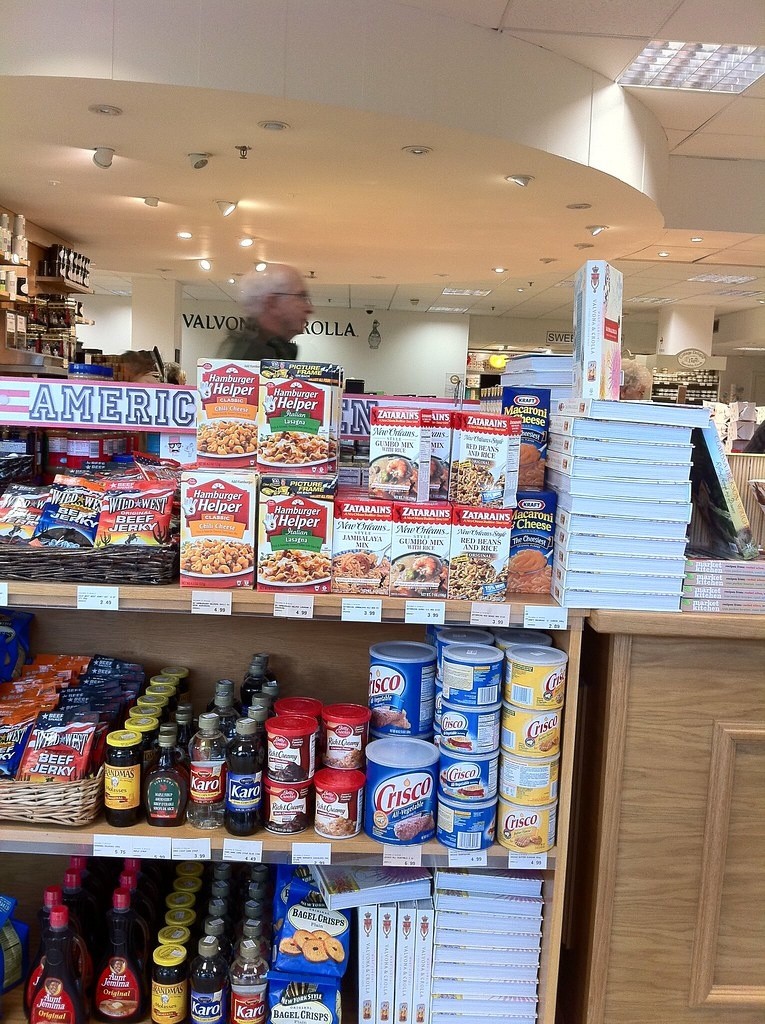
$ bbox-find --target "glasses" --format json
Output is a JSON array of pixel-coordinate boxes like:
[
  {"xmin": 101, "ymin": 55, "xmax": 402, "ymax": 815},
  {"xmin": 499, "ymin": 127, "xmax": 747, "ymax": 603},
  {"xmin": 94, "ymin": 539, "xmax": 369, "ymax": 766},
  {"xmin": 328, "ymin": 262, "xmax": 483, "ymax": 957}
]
[{"xmin": 272, "ymin": 289, "xmax": 312, "ymax": 303}]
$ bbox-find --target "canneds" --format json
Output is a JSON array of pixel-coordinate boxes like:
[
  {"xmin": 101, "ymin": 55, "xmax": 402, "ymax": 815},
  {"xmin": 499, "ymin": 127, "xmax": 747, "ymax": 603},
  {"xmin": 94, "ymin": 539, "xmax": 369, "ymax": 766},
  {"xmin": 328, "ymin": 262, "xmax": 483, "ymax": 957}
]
[
  {"xmin": 261, "ymin": 624, "xmax": 569, "ymax": 854},
  {"xmin": 149, "ymin": 860, "xmax": 204, "ymax": 1024},
  {"xmin": 0, "ymin": 213, "xmax": 91, "ymax": 299},
  {"xmin": 105, "ymin": 666, "xmax": 191, "ymax": 827}
]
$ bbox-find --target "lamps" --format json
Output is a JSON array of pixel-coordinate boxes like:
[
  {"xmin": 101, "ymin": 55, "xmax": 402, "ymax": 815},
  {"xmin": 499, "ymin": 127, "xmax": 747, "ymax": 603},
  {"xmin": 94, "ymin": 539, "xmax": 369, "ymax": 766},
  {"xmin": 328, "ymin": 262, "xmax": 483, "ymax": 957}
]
[
  {"xmin": 505, "ymin": 174, "xmax": 535, "ymax": 187},
  {"xmin": 255, "ymin": 263, "xmax": 267, "ymax": 272},
  {"xmin": 200, "ymin": 259, "xmax": 214, "ymax": 270},
  {"xmin": 587, "ymin": 225, "xmax": 609, "ymax": 236},
  {"xmin": 92, "ymin": 147, "xmax": 115, "ymax": 169},
  {"xmin": 217, "ymin": 202, "xmax": 237, "ymax": 217},
  {"xmin": 144, "ymin": 197, "xmax": 159, "ymax": 207},
  {"xmin": 188, "ymin": 153, "xmax": 209, "ymax": 170}
]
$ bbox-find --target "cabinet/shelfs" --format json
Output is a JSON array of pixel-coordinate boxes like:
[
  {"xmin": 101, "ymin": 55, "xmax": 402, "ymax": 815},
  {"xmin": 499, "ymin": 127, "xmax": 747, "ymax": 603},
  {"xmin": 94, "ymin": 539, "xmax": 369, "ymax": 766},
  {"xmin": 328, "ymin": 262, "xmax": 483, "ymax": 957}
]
[
  {"xmin": 0, "ymin": 252, "xmax": 95, "ymax": 380},
  {"xmin": 634, "ymin": 349, "xmax": 726, "ymax": 405},
  {"xmin": 0, "ymin": 580, "xmax": 590, "ymax": 1024},
  {"xmin": 566, "ymin": 610, "xmax": 764, "ymax": 1023},
  {"xmin": 466, "ymin": 349, "xmax": 550, "ymax": 387}
]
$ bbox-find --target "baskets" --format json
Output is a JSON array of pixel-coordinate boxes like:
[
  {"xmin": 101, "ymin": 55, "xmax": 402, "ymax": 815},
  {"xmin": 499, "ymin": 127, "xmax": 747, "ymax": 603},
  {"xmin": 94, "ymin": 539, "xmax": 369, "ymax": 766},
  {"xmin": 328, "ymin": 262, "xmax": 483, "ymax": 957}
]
[
  {"xmin": 1, "ymin": 773, "xmax": 107, "ymax": 827},
  {"xmin": 0, "ymin": 537, "xmax": 174, "ymax": 586}
]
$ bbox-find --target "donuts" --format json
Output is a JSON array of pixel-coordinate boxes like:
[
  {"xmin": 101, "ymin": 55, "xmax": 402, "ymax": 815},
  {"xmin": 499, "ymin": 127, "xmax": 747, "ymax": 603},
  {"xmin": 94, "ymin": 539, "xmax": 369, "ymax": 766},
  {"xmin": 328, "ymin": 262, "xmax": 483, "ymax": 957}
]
[{"xmin": 279, "ymin": 929, "xmax": 345, "ymax": 963}]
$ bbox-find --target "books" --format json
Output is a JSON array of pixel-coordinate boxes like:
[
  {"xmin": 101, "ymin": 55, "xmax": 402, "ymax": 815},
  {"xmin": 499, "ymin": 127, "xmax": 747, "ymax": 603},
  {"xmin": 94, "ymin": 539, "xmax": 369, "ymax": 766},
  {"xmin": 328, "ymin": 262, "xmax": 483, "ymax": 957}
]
[{"xmin": 498, "ymin": 352, "xmax": 765, "ymax": 615}]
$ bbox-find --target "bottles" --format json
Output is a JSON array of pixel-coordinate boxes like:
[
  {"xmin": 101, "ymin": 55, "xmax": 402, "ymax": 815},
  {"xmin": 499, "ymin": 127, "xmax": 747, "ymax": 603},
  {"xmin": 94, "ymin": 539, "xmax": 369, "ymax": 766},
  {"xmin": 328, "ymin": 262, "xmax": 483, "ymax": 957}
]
[
  {"xmin": 23, "ymin": 854, "xmax": 273, "ymax": 1024},
  {"xmin": 143, "ymin": 652, "xmax": 279, "ymax": 836}
]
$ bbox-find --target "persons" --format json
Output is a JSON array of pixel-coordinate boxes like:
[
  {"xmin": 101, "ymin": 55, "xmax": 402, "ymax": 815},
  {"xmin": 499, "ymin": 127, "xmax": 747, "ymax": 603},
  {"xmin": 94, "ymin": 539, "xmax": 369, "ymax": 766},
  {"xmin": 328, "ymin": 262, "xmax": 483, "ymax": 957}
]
[
  {"xmin": 119, "ymin": 350, "xmax": 187, "ymax": 385},
  {"xmin": 742, "ymin": 419, "xmax": 765, "ymax": 454},
  {"xmin": 212, "ymin": 262, "xmax": 315, "ymax": 360}
]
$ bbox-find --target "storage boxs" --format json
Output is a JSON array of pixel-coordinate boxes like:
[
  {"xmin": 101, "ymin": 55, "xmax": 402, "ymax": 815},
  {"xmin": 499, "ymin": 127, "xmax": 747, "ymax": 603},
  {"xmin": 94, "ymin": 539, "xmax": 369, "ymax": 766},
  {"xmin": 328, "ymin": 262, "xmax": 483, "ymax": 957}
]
[
  {"xmin": 703, "ymin": 399, "xmax": 765, "ymax": 454},
  {"xmin": 180, "ymin": 358, "xmax": 559, "ymax": 602}
]
